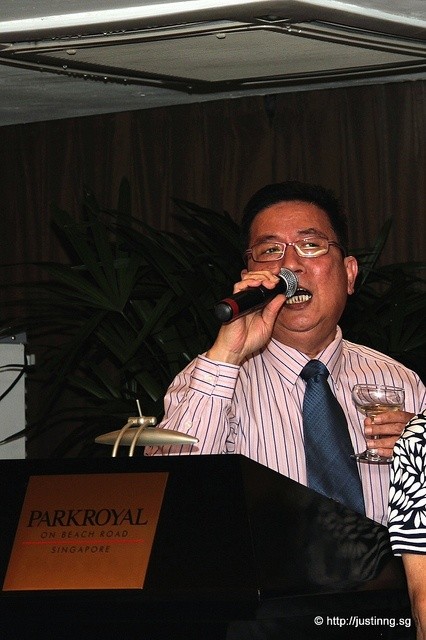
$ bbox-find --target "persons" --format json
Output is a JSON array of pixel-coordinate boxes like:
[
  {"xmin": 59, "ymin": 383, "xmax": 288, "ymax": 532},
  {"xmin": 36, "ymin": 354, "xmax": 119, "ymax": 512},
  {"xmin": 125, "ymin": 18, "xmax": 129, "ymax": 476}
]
[
  {"xmin": 388, "ymin": 410, "xmax": 425, "ymax": 640},
  {"xmin": 143, "ymin": 182, "xmax": 425, "ymax": 528}
]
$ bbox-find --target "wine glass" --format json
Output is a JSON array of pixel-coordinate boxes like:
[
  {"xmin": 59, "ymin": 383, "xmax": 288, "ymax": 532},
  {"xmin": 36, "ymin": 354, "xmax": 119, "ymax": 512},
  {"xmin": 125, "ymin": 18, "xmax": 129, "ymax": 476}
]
[{"xmin": 351, "ymin": 383, "xmax": 407, "ymax": 465}]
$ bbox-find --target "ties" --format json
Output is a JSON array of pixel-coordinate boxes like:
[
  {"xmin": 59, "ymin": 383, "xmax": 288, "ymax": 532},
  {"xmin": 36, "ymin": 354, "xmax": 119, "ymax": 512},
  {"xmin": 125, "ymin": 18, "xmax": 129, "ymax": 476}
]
[{"xmin": 300, "ymin": 360, "xmax": 365, "ymax": 515}]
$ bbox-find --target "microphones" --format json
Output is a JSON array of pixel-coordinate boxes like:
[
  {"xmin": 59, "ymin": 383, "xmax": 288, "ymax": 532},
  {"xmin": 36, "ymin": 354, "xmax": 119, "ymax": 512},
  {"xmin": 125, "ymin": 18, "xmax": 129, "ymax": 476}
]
[{"xmin": 212, "ymin": 267, "xmax": 299, "ymax": 326}]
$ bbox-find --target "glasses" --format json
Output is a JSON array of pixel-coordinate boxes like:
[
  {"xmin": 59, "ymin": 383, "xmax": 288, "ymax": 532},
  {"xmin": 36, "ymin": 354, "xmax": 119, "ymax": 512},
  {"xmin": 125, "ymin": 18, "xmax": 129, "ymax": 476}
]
[{"xmin": 242, "ymin": 236, "xmax": 347, "ymax": 262}]
